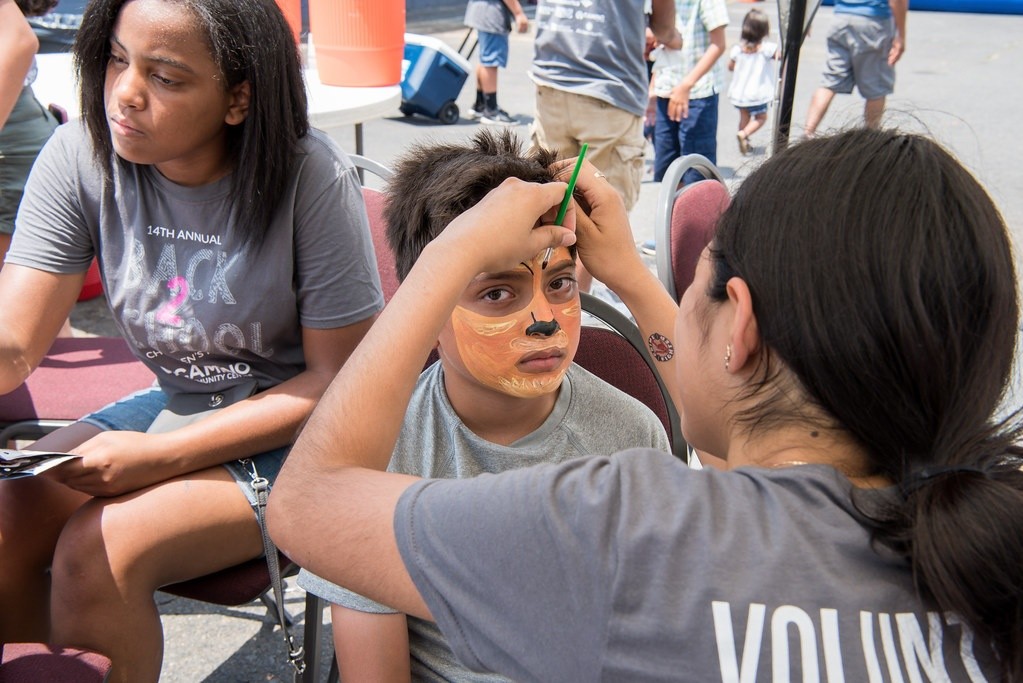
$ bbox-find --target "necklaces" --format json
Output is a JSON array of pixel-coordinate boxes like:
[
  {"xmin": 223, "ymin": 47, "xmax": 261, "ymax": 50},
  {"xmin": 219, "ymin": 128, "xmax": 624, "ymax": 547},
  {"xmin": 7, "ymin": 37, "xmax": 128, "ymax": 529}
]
[{"xmin": 771, "ymin": 459, "xmax": 809, "ymax": 469}]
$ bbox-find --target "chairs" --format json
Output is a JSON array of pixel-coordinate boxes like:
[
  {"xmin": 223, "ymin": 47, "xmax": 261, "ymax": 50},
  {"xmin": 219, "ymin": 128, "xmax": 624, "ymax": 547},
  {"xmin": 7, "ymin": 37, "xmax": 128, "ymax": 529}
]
[
  {"xmin": 419, "ymin": 288, "xmax": 691, "ymax": 465},
  {"xmin": 654, "ymin": 153, "xmax": 732, "ymax": 305},
  {"xmin": 0, "ymin": 290, "xmax": 160, "ymax": 448},
  {"xmin": 153, "ymin": 153, "xmax": 403, "ymax": 683}
]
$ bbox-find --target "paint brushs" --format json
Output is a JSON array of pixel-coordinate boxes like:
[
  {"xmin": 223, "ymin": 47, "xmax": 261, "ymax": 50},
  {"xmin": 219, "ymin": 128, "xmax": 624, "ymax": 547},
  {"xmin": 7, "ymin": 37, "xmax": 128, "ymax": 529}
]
[{"xmin": 542, "ymin": 143, "xmax": 588, "ymax": 271}]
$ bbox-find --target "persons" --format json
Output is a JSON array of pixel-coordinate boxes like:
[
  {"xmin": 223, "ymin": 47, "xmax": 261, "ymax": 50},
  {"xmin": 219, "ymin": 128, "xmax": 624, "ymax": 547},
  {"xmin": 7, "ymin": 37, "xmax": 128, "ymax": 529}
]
[
  {"xmin": 465, "ymin": 0, "xmax": 909, "ymax": 292},
  {"xmin": 263, "ymin": 128, "xmax": 1022, "ymax": 683},
  {"xmin": 0, "ymin": 0, "xmax": 385, "ymax": 683},
  {"xmin": 0, "ymin": 0, "xmax": 61, "ymax": 273},
  {"xmin": 295, "ymin": 128, "xmax": 674, "ymax": 683}
]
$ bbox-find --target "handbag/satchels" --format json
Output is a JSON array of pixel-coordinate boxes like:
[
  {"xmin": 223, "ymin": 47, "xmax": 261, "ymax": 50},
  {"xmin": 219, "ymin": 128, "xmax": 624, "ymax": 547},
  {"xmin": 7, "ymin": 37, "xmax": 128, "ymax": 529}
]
[{"xmin": 145, "ymin": 379, "xmax": 258, "ymax": 434}]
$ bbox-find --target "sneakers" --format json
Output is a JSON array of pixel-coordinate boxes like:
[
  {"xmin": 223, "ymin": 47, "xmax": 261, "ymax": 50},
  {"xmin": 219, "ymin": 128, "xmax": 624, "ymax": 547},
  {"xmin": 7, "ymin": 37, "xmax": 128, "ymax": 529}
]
[
  {"xmin": 468, "ymin": 103, "xmax": 484, "ymax": 116},
  {"xmin": 480, "ymin": 109, "xmax": 520, "ymax": 125}
]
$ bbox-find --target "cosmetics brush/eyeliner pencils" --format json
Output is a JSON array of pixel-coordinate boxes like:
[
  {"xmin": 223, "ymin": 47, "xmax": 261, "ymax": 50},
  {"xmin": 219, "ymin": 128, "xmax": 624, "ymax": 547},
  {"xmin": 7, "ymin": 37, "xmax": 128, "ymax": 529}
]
[{"xmin": 541, "ymin": 142, "xmax": 588, "ymax": 268}]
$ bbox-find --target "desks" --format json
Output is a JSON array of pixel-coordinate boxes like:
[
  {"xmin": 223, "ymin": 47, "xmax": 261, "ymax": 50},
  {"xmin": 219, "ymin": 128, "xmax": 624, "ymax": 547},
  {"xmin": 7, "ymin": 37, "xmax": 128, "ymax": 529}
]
[{"xmin": 298, "ymin": 44, "xmax": 403, "ymax": 186}]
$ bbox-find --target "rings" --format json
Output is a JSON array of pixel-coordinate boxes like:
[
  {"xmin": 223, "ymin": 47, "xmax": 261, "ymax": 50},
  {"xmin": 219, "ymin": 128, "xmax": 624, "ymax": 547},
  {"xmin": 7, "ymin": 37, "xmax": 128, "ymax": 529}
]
[{"xmin": 593, "ymin": 170, "xmax": 606, "ymax": 180}]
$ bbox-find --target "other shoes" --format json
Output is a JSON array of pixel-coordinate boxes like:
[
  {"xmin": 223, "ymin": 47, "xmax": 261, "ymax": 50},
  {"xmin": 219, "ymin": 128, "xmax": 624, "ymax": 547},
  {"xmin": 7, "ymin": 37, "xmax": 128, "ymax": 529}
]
[
  {"xmin": 641, "ymin": 240, "xmax": 656, "ymax": 256},
  {"xmin": 745, "ymin": 138, "xmax": 752, "ymax": 152},
  {"xmin": 736, "ymin": 130, "xmax": 748, "ymax": 155}
]
